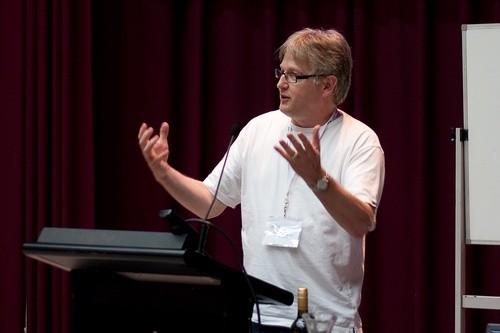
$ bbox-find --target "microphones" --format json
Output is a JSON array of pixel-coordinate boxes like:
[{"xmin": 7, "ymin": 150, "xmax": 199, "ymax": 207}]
[{"xmin": 194, "ymin": 123, "xmax": 242, "ymax": 256}]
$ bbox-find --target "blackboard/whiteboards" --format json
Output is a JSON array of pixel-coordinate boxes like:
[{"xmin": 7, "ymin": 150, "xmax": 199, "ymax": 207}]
[{"xmin": 461, "ymin": 23, "xmax": 499, "ymax": 246}]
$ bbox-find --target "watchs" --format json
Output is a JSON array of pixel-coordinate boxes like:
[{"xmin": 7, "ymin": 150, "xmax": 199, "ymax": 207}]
[{"xmin": 309, "ymin": 172, "xmax": 330, "ymax": 193}]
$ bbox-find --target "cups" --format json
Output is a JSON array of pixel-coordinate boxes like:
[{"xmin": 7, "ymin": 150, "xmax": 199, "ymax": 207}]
[{"xmin": 302, "ymin": 312, "xmax": 337, "ymax": 333}]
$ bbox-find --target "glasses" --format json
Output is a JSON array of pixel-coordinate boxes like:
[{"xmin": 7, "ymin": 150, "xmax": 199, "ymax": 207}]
[{"xmin": 273, "ymin": 67, "xmax": 326, "ymax": 84}]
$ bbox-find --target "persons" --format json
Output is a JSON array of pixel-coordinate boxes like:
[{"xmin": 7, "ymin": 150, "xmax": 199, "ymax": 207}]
[{"xmin": 137, "ymin": 27, "xmax": 386, "ymax": 333}]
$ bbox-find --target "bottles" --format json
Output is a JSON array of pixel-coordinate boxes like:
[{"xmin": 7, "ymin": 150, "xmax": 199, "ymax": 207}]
[{"xmin": 290, "ymin": 288, "xmax": 315, "ymax": 333}]
